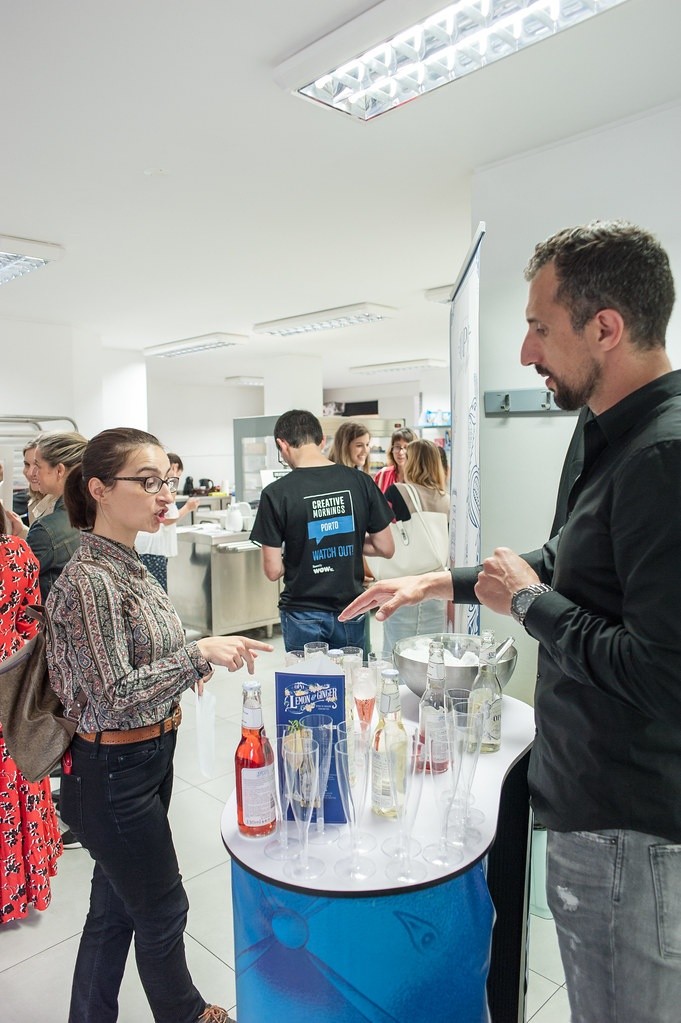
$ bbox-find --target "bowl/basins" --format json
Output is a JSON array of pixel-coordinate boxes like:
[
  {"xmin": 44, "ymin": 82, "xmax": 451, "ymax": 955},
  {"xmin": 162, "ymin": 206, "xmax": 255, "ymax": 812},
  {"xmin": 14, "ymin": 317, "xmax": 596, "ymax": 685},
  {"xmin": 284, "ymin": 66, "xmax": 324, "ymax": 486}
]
[{"xmin": 395, "ymin": 633, "xmax": 518, "ymax": 712}]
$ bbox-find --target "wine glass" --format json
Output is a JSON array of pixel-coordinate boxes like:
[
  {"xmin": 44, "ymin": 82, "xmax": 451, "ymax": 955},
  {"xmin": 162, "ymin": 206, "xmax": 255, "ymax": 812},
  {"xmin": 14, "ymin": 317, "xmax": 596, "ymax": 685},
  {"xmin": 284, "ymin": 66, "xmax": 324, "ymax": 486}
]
[{"xmin": 260, "ymin": 641, "xmax": 485, "ymax": 865}]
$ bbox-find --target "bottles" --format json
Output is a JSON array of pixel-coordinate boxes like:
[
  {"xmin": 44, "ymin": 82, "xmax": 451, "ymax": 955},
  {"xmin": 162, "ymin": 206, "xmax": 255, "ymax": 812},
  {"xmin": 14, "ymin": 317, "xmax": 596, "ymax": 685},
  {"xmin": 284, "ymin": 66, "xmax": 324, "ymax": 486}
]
[
  {"xmin": 467, "ymin": 628, "xmax": 503, "ymax": 751},
  {"xmin": 418, "ymin": 641, "xmax": 452, "ymax": 775},
  {"xmin": 183, "ymin": 476, "xmax": 194, "ymax": 495},
  {"xmin": 235, "ymin": 680, "xmax": 277, "ymax": 840}
]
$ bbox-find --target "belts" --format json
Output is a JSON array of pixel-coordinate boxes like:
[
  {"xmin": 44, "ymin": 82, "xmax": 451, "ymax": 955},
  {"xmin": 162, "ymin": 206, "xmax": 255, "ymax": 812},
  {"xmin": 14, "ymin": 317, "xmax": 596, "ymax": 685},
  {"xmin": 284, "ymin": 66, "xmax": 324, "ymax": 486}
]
[{"xmin": 77, "ymin": 705, "xmax": 182, "ymax": 744}]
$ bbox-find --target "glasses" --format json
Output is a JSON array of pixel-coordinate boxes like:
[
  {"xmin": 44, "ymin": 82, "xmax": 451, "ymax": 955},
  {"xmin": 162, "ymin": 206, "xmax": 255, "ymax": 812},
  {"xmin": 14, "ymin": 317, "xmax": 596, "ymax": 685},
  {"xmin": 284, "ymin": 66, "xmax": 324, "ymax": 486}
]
[
  {"xmin": 113, "ymin": 476, "xmax": 179, "ymax": 494},
  {"xmin": 277, "ymin": 446, "xmax": 289, "ymax": 467},
  {"xmin": 392, "ymin": 445, "xmax": 407, "ymax": 454}
]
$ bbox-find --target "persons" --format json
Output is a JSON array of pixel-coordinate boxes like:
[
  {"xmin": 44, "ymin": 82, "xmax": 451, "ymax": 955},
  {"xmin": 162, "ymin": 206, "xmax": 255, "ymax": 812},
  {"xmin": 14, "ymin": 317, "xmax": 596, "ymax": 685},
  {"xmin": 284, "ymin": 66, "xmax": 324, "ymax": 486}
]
[
  {"xmin": 249, "ymin": 408, "xmax": 397, "ymax": 662},
  {"xmin": 133, "ymin": 452, "xmax": 200, "ymax": 594},
  {"xmin": 337, "ymin": 221, "xmax": 681, "ymax": 1023},
  {"xmin": 42, "ymin": 426, "xmax": 274, "ymax": 1023},
  {"xmin": 0, "ymin": 430, "xmax": 94, "ymax": 928},
  {"xmin": 327, "ymin": 422, "xmax": 450, "ymax": 667}
]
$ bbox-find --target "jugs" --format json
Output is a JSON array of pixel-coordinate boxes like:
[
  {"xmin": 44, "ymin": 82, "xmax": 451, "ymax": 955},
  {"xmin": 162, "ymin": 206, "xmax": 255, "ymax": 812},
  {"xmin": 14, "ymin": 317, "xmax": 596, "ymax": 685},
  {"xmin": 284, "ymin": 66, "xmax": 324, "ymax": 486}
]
[{"xmin": 199, "ymin": 479, "xmax": 213, "ymax": 490}]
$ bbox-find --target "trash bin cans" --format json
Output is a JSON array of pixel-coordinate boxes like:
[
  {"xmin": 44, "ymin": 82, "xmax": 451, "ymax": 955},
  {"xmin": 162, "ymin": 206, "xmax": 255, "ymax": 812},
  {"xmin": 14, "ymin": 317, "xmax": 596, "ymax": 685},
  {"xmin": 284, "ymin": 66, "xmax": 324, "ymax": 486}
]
[{"xmin": 529, "ymin": 823, "xmax": 556, "ymax": 919}]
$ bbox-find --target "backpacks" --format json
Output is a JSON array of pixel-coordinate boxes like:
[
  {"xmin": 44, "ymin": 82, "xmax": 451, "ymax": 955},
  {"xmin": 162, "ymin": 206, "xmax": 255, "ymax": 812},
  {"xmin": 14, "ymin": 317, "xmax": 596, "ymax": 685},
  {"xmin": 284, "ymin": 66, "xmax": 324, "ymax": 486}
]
[{"xmin": 0, "ymin": 562, "xmax": 119, "ymax": 783}]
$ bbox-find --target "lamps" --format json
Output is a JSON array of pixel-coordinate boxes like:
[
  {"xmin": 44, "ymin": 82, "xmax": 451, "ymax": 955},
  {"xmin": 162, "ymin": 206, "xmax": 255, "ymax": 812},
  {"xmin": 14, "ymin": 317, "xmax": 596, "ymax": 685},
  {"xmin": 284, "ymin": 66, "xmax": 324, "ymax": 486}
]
[
  {"xmin": 271, "ymin": 0, "xmax": 629, "ymax": 123},
  {"xmin": 425, "ymin": 284, "xmax": 454, "ymax": 305},
  {"xmin": 224, "ymin": 376, "xmax": 263, "ymax": 386},
  {"xmin": 0, "ymin": 236, "xmax": 63, "ymax": 285},
  {"xmin": 252, "ymin": 302, "xmax": 399, "ymax": 342},
  {"xmin": 351, "ymin": 359, "xmax": 450, "ymax": 376},
  {"xmin": 143, "ymin": 333, "xmax": 249, "ymax": 358}
]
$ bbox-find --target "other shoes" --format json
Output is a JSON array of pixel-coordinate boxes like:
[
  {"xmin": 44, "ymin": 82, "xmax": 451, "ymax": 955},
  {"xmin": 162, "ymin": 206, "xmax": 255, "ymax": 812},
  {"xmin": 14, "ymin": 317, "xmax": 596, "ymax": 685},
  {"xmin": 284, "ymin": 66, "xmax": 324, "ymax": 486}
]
[
  {"xmin": 50, "ymin": 788, "xmax": 60, "ymax": 801},
  {"xmin": 55, "ymin": 802, "xmax": 59, "ymax": 817},
  {"xmin": 200, "ymin": 1002, "xmax": 235, "ymax": 1022},
  {"xmin": 60, "ymin": 829, "xmax": 82, "ymax": 849}
]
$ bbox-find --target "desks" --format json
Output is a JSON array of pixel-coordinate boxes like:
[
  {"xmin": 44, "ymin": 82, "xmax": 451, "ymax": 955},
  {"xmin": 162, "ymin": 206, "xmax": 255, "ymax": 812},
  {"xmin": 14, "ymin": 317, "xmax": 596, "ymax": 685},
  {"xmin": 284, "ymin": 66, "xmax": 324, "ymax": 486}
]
[{"xmin": 221, "ymin": 690, "xmax": 535, "ymax": 1023}]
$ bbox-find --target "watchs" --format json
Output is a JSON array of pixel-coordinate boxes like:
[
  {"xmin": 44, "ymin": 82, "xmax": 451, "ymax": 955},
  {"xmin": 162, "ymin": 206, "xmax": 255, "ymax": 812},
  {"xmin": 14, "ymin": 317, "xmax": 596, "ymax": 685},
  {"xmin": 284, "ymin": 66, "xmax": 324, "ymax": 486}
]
[{"xmin": 510, "ymin": 583, "xmax": 554, "ymax": 627}]
